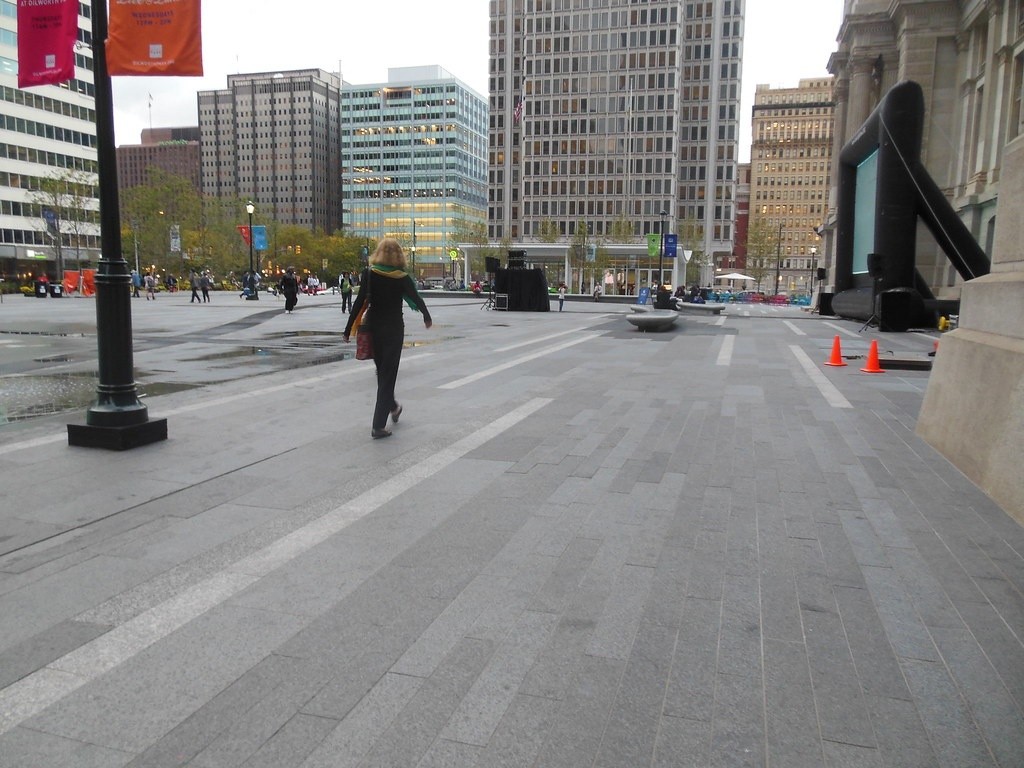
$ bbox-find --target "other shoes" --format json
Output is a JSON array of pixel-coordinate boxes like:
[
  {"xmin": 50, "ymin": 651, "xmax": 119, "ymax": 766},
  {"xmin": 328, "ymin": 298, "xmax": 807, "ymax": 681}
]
[
  {"xmin": 372, "ymin": 429, "xmax": 392, "ymax": 438},
  {"xmin": 392, "ymin": 405, "xmax": 402, "ymax": 423}
]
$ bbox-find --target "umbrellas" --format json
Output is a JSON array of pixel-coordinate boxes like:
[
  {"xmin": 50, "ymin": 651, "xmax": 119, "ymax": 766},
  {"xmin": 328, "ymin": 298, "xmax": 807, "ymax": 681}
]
[{"xmin": 714, "ymin": 273, "xmax": 756, "ymax": 294}]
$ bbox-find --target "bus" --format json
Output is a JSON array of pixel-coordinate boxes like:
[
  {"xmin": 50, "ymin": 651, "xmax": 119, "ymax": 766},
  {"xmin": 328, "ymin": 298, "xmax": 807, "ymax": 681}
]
[{"xmin": 425, "ymin": 277, "xmax": 464, "ymax": 290}]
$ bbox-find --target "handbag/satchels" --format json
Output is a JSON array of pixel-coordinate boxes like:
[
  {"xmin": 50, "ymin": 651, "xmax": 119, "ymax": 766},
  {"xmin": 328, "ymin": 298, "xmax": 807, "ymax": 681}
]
[
  {"xmin": 356, "ymin": 325, "xmax": 375, "ymax": 360},
  {"xmin": 148, "ymin": 277, "xmax": 155, "ymax": 288}
]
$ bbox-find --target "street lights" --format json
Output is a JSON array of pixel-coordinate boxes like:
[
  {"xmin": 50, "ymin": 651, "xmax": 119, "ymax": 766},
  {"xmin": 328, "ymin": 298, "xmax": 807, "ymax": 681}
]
[
  {"xmin": 658, "ymin": 209, "xmax": 668, "ymax": 286},
  {"xmin": 810, "ymin": 247, "xmax": 817, "ymax": 298},
  {"xmin": 245, "ymin": 200, "xmax": 255, "ymax": 277},
  {"xmin": 134, "ymin": 211, "xmax": 164, "ymax": 274},
  {"xmin": 775, "ymin": 224, "xmax": 787, "ymax": 295},
  {"xmin": 359, "ymin": 239, "xmax": 376, "ymax": 266},
  {"xmin": 413, "ymin": 220, "xmax": 422, "ymax": 274},
  {"xmin": 579, "ymin": 224, "xmax": 592, "ymax": 294}
]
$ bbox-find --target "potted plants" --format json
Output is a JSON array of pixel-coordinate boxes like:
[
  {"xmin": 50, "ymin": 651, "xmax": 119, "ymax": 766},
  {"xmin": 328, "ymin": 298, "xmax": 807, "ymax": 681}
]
[{"xmin": 20, "ymin": 285, "xmax": 36, "ymax": 296}]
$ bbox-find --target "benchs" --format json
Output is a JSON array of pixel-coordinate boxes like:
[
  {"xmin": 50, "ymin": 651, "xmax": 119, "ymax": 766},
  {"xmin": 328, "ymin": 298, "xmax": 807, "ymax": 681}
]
[
  {"xmin": 626, "ymin": 306, "xmax": 677, "ymax": 332},
  {"xmin": 676, "ymin": 301, "xmax": 726, "ymax": 316}
]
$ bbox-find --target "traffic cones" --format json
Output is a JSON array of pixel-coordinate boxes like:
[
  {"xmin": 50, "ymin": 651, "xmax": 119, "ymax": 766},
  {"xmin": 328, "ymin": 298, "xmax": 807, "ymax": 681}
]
[
  {"xmin": 858, "ymin": 339, "xmax": 887, "ymax": 373},
  {"xmin": 824, "ymin": 335, "xmax": 848, "ymax": 366}
]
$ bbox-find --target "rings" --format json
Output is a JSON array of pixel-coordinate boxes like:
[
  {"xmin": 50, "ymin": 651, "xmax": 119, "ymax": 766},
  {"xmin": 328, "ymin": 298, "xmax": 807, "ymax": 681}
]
[{"xmin": 344, "ymin": 339, "xmax": 346, "ymax": 341}]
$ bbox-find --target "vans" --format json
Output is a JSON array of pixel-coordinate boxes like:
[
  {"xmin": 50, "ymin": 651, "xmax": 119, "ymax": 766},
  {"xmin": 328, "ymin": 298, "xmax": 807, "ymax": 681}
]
[{"xmin": 739, "ymin": 290, "xmax": 765, "ymax": 296}]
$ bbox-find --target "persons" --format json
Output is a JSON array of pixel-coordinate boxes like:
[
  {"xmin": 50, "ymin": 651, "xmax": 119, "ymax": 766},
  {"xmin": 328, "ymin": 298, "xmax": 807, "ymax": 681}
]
[
  {"xmin": 132, "ymin": 267, "xmax": 362, "ymax": 314},
  {"xmin": 621, "ymin": 285, "xmax": 748, "ymax": 310},
  {"xmin": 418, "ymin": 278, "xmax": 483, "ymax": 299},
  {"xmin": 342, "ymin": 238, "xmax": 432, "ymax": 437},
  {"xmin": 593, "ymin": 282, "xmax": 603, "ymax": 302},
  {"xmin": 558, "ymin": 283, "xmax": 565, "ymax": 312}
]
